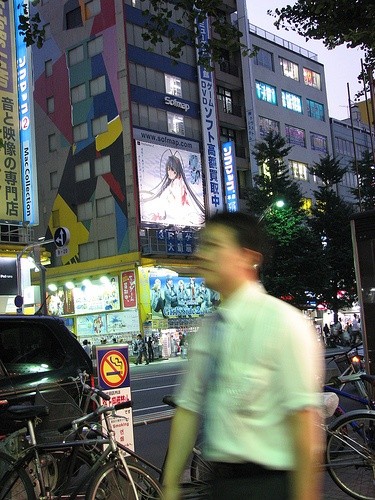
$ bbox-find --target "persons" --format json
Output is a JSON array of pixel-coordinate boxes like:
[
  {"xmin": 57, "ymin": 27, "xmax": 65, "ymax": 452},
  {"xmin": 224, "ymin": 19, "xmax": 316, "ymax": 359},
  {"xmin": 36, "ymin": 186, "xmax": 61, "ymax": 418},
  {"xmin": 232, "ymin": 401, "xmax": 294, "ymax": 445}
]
[
  {"xmin": 82, "ymin": 329, "xmax": 185, "ymax": 365},
  {"xmin": 159, "ymin": 211, "xmax": 328, "ymax": 500},
  {"xmin": 314, "ymin": 313, "xmax": 363, "ymax": 349}
]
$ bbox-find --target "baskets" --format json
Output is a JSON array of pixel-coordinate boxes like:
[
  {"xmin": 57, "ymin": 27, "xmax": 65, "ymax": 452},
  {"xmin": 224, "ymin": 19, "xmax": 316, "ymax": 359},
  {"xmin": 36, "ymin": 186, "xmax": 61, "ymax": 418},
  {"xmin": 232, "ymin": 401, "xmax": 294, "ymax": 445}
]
[
  {"xmin": 336, "ymin": 350, "xmax": 363, "ymax": 376},
  {"xmin": 31, "ymin": 382, "xmax": 84, "ymax": 441}
]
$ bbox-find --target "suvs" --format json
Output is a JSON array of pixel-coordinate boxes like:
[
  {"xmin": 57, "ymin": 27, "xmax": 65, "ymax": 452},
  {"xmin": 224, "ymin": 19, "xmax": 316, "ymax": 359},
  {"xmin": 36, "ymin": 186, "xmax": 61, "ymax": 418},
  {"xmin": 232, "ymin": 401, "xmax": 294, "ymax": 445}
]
[{"xmin": 0, "ymin": 314, "xmax": 100, "ymax": 442}]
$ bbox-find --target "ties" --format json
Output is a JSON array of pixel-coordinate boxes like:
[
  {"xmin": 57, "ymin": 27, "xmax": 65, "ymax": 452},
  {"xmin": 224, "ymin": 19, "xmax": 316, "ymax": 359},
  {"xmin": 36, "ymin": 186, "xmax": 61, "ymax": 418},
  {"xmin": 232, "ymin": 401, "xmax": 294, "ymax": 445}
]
[{"xmin": 200, "ymin": 309, "xmax": 225, "ymax": 452}]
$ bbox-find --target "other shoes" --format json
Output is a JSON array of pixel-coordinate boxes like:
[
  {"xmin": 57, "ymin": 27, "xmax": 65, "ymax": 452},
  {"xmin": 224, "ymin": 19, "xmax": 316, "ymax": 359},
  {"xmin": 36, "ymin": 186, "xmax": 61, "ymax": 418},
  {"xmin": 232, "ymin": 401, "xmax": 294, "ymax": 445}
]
[
  {"xmin": 145, "ymin": 362, "xmax": 149, "ymax": 365},
  {"xmin": 134, "ymin": 362, "xmax": 138, "ymax": 365},
  {"xmin": 139, "ymin": 363, "xmax": 142, "ymax": 364}
]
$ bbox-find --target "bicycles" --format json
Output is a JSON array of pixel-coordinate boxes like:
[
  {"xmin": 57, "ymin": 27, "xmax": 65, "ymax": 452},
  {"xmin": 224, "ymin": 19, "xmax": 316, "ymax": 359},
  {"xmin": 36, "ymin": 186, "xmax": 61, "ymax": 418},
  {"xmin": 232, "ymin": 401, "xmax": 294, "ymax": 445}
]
[
  {"xmin": 0, "ymin": 368, "xmax": 217, "ymax": 500},
  {"xmin": 323, "ymin": 341, "xmax": 375, "ymax": 500}
]
